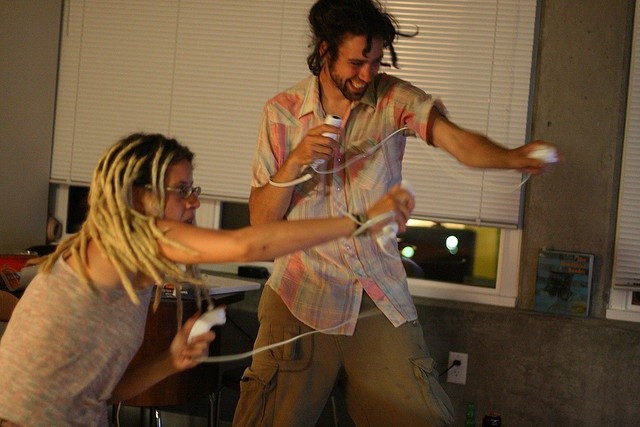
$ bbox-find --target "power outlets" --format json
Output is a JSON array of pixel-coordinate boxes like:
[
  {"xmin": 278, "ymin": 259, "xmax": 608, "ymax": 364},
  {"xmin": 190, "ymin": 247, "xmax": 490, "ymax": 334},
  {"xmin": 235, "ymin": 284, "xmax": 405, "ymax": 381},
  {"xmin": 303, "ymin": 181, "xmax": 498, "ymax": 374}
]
[{"xmin": 445, "ymin": 351, "xmax": 469, "ymax": 384}]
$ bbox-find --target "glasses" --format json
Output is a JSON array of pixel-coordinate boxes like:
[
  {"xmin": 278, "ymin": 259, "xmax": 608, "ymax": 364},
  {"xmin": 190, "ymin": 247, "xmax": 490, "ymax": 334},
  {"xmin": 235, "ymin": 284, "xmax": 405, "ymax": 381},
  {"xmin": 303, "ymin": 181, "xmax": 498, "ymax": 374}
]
[{"xmin": 139, "ymin": 184, "xmax": 201, "ymax": 200}]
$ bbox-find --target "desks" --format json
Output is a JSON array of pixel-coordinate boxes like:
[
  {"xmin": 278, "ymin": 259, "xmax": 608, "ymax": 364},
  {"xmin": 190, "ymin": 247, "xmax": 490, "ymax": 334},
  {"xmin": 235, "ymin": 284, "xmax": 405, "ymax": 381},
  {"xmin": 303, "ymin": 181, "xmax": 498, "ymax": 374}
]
[{"xmin": 1, "ymin": 256, "xmax": 267, "ymax": 427}]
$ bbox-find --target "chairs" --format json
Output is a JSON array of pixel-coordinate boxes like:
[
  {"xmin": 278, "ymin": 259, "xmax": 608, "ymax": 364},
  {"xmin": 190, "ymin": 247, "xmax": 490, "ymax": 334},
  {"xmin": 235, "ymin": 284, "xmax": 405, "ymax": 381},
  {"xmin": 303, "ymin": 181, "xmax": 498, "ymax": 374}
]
[{"xmin": 107, "ymin": 305, "xmax": 217, "ymax": 427}]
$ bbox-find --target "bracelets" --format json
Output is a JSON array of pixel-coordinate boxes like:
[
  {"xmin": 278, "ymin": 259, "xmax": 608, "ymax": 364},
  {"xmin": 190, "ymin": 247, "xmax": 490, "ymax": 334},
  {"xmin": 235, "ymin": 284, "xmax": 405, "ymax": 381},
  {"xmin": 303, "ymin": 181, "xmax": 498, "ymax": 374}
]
[{"xmin": 353, "ymin": 213, "xmax": 368, "ymax": 236}]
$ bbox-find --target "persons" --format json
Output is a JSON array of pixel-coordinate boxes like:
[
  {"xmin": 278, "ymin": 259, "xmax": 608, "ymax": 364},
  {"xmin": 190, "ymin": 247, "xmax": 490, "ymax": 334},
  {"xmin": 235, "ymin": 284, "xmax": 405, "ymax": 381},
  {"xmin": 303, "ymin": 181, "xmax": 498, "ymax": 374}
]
[
  {"xmin": 231, "ymin": 0, "xmax": 567, "ymax": 427},
  {"xmin": 0, "ymin": 132, "xmax": 417, "ymax": 427}
]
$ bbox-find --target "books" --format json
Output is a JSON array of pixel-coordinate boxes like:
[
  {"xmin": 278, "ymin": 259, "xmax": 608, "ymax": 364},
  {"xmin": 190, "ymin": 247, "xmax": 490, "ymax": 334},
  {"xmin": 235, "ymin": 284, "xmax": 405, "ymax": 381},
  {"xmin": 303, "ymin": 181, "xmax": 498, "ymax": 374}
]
[{"xmin": 533, "ymin": 248, "xmax": 595, "ymax": 318}]
